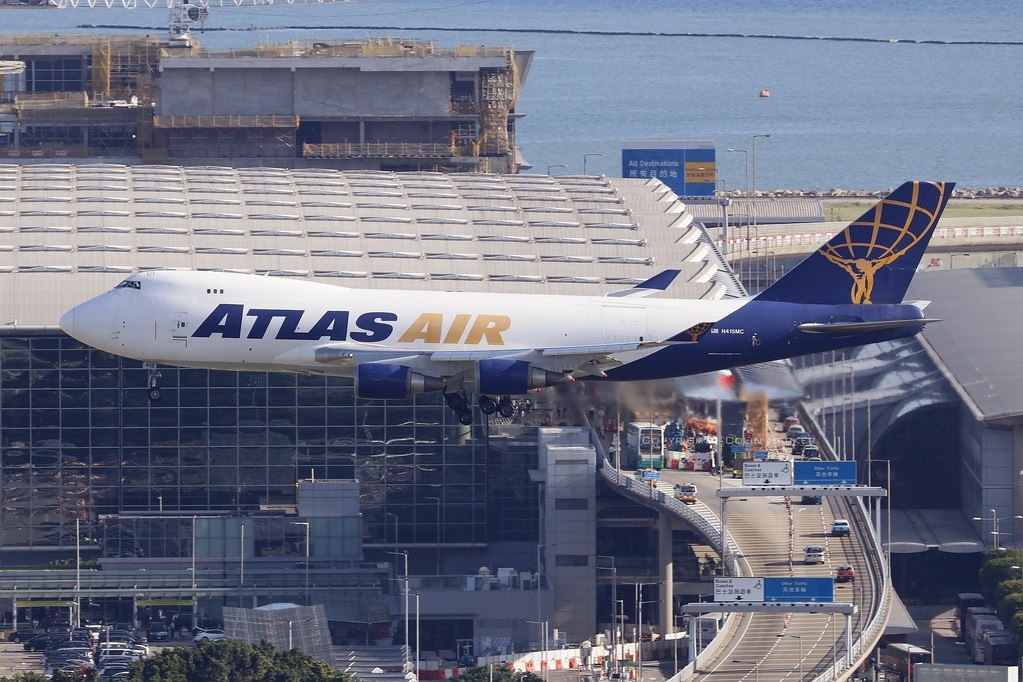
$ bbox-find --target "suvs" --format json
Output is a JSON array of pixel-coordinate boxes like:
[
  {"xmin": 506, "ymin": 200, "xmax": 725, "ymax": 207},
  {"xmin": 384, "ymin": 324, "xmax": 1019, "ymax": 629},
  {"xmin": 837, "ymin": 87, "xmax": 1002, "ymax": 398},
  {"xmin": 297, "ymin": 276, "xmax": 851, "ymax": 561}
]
[
  {"xmin": 633, "ymin": 468, "xmax": 661, "ymax": 488},
  {"xmin": 673, "ymin": 482, "xmax": 698, "ymax": 504},
  {"xmin": 147, "ymin": 622, "xmax": 169, "ymax": 642}
]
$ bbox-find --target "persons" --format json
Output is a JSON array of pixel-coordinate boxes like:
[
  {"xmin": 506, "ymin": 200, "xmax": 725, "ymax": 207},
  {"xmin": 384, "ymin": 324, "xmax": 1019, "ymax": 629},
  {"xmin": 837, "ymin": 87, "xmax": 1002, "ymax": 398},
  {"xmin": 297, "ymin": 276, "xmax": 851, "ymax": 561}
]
[
  {"xmin": 607, "ymin": 444, "xmax": 614, "ymax": 463},
  {"xmin": 169, "ymin": 621, "xmax": 174, "ymax": 639}
]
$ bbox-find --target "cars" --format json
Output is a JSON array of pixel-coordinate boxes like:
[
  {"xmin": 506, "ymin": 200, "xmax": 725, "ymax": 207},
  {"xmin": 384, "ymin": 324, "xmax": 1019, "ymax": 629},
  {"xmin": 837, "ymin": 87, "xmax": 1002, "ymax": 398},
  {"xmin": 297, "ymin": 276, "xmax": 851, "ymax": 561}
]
[
  {"xmin": 791, "ymin": 432, "xmax": 814, "ymax": 455},
  {"xmin": 801, "ymin": 445, "xmax": 821, "ymax": 460},
  {"xmin": 194, "ymin": 629, "xmax": 226, "ymax": 642},
  {"xmin": 783, "ymin": 416, "xmax": 800, "ymax": 432},
  {"xmin": 803, "ymin": 544, "xmax": 825, "ymax": 564},
  {"xmin": 835, "ymin": 563, "xmax": 855, "ymax": 582},
  {"xmin": 807, "ymin": 457, "xmax": 822, "ymax": 462},
  {"xmin": 786, "ymin": 425, "xmax": 805, "ymax": 438},
  {"xmin": 7, "ymin": 629, "xmax": 150, "ymax": 682},
  {"xmin": 802, "ymin": 496, "xmax": 822, "ymax": 505},
  {"xmin": 830, "ymin": 519, "xmax": 850, "ymax": 536}
]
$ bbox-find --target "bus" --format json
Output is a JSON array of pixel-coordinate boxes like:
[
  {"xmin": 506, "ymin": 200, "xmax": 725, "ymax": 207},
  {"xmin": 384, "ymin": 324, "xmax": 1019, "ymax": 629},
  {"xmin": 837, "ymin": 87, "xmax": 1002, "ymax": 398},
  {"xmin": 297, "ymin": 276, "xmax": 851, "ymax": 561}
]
[
  {"xmin": 982, "ymin": 629, "xmax": 1018, "ymax": 668},
  {"xmin": 965, "ymin": 606, "xmax": 993, "ymax": 646},
  {"xmin": 883, "ymin": 643, "xmax": 932, "ymax": 682},
  {"xmin": 968, "ymin": 614, "xmax": 1004, "ymax": 665},
  {"xmin": 954, "ymin": 593, "xmax": 985, "ymax": 640}
]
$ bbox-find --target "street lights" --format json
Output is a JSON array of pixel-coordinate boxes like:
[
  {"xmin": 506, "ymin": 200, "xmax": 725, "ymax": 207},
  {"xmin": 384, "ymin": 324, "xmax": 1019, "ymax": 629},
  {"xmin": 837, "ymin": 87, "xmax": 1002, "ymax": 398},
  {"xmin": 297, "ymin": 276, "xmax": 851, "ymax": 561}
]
[
  {"xmin": 548, "ymin": 164, "xmax": 568, "ymax": 174},
  {"xmin": 699, "ymin": 133, "xmax": 785, "ymax": 294},
  {"xmin": 288, "ymin": 352, "xmax": 1023, "ymax": 682},
  {"xmin": 583, "ymin": 153, "xmax": 606, "ymax": 174}
]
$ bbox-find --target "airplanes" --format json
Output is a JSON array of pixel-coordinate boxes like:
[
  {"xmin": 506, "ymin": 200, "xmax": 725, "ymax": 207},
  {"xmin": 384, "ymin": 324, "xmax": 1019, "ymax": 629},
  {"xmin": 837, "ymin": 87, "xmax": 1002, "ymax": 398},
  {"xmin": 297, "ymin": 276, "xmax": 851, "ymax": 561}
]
[{"xmin": 55, "ymin": 180, "xmax": 959, "ymax": 427}]
